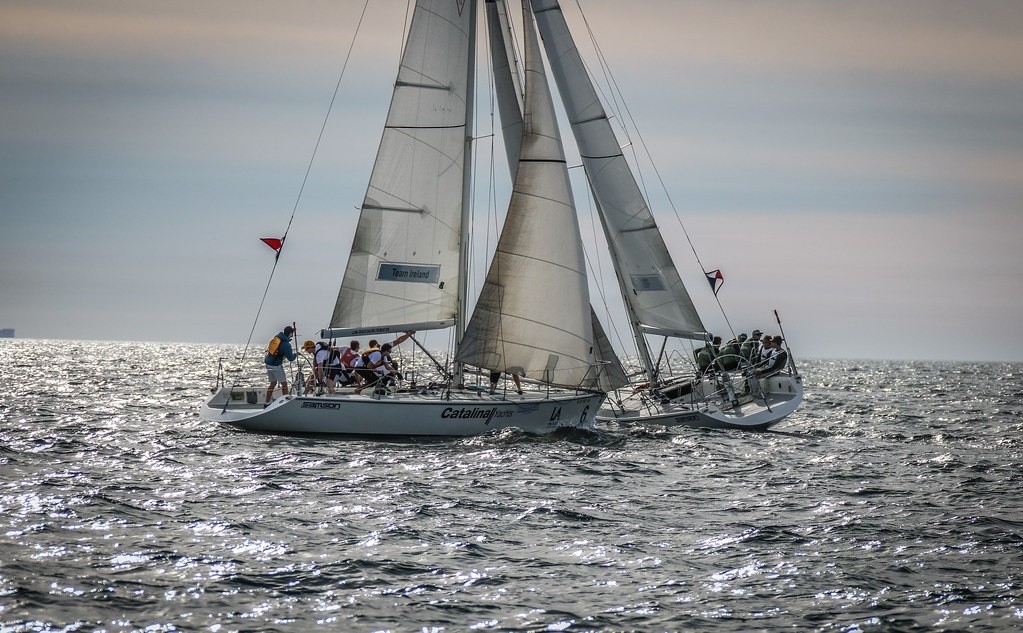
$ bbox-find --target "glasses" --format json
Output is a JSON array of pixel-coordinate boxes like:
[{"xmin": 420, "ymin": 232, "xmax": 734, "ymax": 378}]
[
  {"xmin": 755, "ymin": 333, "xmax": 761, "ymax": 336},
  {"xmin": 387, "ymin": 350, "xmax": 391, "ymax": 353}
]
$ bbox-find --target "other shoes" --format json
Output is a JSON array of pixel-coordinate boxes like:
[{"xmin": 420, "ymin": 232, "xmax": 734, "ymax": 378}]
[
  {"xmin": 752, "ymin": 391, "xmax": 757, "ymax": 396},
  {"xmin": 264, "ymin": 403, "xmax": 271, "ymax": 409},
  {"xmin": 748, "ymin": 391, "xmax": 752, "ymax": 395}
]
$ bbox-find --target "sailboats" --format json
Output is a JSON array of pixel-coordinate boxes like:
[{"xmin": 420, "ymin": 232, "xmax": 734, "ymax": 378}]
[
  {"xmin": 518, "ymin": 0, "xmax": 806, "ymax": 428},
  {"xmin": 201, "ymin": 0, "xmax": 636, "ymax": 452}
]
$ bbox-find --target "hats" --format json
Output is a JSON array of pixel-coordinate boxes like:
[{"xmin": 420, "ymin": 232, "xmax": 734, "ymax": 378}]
[
  {"xmin": 769, "ymin": 336, "xmax": 782, "ymax": 342},
  {"xmin": 741, "ymin": 333, "xmax": 747, "ymax": 338},
  {"xmin": 753, "ymin": 329, "xmax": 763, "ymax": 334},
  {"xmin": 284, "ymin": 326, "xmax": 296, "ymax": 333},
  {"xmin": 760, "ymin": 335, "xmax": 771, "ymax": 342},
  {"xmin": 301, "ymin": 340, "xmax": 315, "ymax": 348}
]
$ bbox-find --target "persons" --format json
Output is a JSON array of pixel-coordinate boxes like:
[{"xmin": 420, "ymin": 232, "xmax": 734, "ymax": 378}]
[
  {"xmin": 301, "ymin": 341, "xmax": 341, "ymax": 396},
  {"xmin": 490, "ymin": 370, "xmax": 523, "ymax": 395},
  {"xmin": 264, "ymin": 326, "xmax": 297, "ymax": 409},
  {"xmin": 693, "ymin": 330, "xmax": 787, "ymax": 395},
  {"xmin": 335, "ymin": 330, "xmax": 415, "ymax": 395}
]
